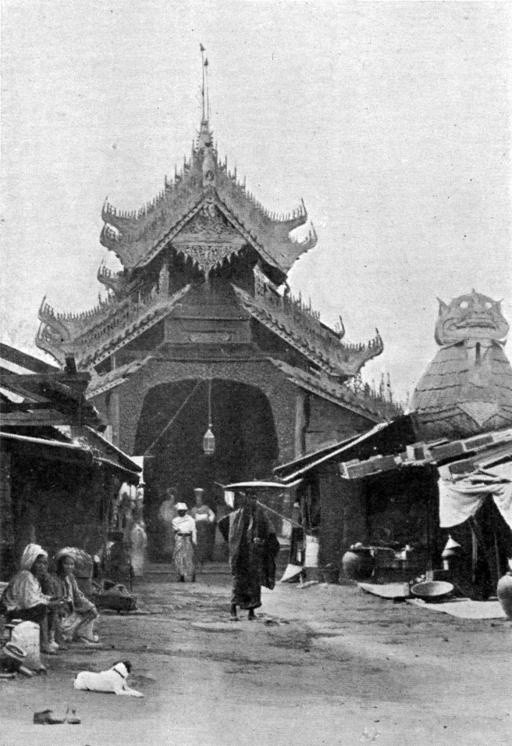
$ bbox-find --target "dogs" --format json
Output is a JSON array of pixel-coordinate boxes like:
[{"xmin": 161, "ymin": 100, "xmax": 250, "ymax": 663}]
[{"xmin": 73, "ymin": 660, "xmax": 145, "ymax": 698}]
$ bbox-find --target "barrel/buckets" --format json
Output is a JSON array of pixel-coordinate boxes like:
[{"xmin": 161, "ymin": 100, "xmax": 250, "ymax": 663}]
[{"xmin": 320, "ymin": 567, "xmax": 340, "ymax": 584}]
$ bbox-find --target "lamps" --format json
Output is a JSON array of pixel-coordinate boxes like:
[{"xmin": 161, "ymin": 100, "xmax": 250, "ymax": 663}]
[{"xmin": 202, "ymin": 377, "xmax": 215, "ymax": 455}]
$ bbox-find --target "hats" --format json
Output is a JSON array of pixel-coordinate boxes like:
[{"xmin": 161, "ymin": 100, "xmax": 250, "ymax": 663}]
[{"xmin": 177, "ymin": 502, "xmax": 192, "ymax": 511}]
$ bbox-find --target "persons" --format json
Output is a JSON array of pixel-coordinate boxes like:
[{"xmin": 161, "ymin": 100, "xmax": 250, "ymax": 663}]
[
  {"xmin": 171, "ymin": 501, "xmax": 198, "ymax": 582},
  {"xmin": 217, "ymin": 494, "xmax": 279, "ymax": 619},
  {"xmin": 188, "ymin": 487, "xmax": 215, "ymax": 563},
  {"xmin": 210, "ymin": 491, "xmax": 234, "ymax": 561},
  {"xmin": 159, "ymin": 487, "xmax": 179, "ymax": 564},
  {"xmin": 43, "ymin": 549, "xmax": 100, "ymax": 649},
  {"xmin": 0, "ymin": 543, "xmax": 67, "ymax": 677}
]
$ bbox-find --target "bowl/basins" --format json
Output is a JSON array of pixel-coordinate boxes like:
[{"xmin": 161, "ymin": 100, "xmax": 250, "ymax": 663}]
[{"xmin": 411, "ymin": 580, "xmax": 455, "ymax": 602}]
